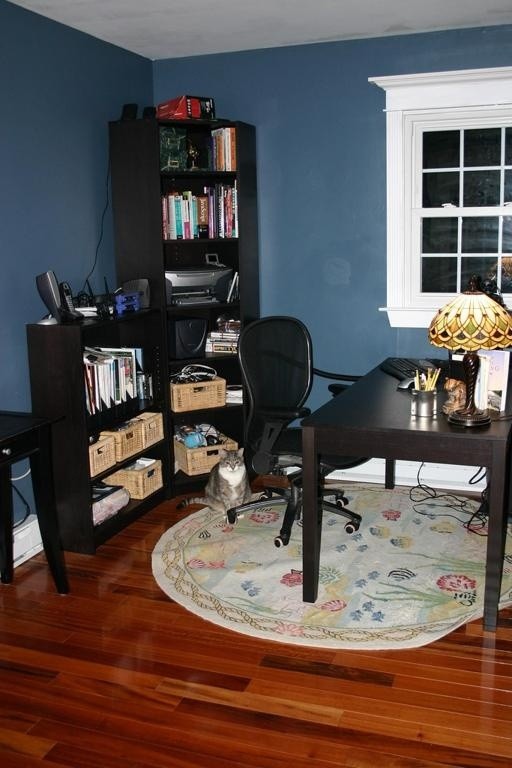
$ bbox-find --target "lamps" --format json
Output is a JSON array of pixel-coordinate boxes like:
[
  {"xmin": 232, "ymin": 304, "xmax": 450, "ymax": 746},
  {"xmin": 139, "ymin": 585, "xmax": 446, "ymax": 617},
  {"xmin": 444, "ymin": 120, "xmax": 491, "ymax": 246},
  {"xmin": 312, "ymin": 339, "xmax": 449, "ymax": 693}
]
[{"xmin": 426, "ymin": 275, "xmax": 511, "ymax": 428}]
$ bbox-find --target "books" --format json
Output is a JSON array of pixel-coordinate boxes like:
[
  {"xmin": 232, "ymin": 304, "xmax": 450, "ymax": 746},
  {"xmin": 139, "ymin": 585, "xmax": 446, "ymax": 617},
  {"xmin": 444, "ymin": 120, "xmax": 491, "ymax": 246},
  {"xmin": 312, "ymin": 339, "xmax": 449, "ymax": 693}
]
[
  {"xmin": 204, "ymin": 330, "xmax": 240, "ymax": 354},
  {"xmin": 83, "ymin": 346, "xmax": 144, "ymax": 416},
  {"xmin": 207, "ymin": 127, "xmax": 237, "ymax": 172},
  {"xmin": 451, "ymin": 349, "xmax": 510, "ymax": 412},
  {"xmin": 162, "ymin": 179, "xmax": 239, "ymax": 241}
]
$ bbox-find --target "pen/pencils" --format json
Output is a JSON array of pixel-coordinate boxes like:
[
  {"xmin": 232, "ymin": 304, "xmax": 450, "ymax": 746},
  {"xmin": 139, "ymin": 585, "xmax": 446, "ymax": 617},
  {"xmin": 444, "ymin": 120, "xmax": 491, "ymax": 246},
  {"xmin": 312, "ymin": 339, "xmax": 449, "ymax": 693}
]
[{"xmin": 416, "ymin": 368, "xmax": 441, "ymax": 391}]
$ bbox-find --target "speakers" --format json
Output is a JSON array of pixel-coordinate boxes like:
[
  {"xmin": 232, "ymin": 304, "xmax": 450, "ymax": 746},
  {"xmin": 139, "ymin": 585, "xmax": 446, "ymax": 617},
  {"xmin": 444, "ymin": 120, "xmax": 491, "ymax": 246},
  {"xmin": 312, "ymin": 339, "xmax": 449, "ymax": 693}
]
[
  {"xmin": 167, "ymin": 316, "xmax": 209, "ymax": 359},
  {"xmin": 34, "ymin": 268, "xmax": 61, "ymax": 325}
]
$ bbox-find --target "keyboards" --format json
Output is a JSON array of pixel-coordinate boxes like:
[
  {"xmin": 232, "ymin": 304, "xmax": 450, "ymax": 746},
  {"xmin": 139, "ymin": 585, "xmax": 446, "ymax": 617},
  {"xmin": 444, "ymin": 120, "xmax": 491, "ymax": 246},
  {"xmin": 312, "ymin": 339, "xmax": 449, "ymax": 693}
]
[{"xmin": 380, "ymin": 356, "xmax": 428, "ymax": 381}]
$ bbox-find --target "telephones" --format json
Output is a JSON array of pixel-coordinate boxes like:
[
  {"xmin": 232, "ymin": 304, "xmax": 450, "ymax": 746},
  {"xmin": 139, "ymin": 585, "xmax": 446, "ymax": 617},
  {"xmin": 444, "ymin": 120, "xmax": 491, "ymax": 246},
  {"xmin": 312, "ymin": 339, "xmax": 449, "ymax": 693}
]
[{"xmin": 57, "ymin": 282, "xmax": 84, "ymax": 322}]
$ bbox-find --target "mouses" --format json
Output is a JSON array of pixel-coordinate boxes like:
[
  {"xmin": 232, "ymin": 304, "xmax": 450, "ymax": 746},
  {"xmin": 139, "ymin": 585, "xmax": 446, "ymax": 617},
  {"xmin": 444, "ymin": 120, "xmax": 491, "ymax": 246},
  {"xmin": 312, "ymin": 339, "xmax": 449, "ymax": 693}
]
[{"xmin": 397, "ymin": 376, "xmax": 415, "ymax": 390}]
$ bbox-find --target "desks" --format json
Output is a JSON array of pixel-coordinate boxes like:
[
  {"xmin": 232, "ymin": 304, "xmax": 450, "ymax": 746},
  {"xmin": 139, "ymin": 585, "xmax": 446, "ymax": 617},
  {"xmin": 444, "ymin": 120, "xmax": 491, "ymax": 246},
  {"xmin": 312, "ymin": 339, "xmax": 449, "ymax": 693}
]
[
  {"xmin": 1, "ymin": 409, "xmax": 71, "ymax": 595},
  {"xmin": 298, "ymin": 354, "xmax": 511, "ymax": 633}
]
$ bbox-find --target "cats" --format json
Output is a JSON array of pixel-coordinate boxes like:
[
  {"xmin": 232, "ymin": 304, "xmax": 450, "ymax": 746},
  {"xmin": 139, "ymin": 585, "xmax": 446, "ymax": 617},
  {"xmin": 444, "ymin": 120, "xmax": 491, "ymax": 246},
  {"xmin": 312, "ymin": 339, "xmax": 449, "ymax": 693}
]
[{"xmin": 175, "ymin": 447, "xmax": 252, "ymax": 520}]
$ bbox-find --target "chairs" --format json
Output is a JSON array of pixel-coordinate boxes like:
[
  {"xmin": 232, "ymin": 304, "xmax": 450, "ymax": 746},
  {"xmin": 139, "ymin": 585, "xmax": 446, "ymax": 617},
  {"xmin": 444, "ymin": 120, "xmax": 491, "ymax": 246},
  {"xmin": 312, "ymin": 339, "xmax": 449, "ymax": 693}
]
[{"xmin": 226, "ymin": 314, "xmax": 367, "ymax": 550}]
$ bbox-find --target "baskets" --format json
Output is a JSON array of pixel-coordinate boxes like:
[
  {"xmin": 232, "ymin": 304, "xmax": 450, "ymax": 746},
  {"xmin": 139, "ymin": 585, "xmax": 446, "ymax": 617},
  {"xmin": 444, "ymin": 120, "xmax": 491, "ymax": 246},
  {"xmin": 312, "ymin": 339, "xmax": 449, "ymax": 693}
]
[{"xmin": 88, "ymin": 412, "xmax": 164, "ymax": 477}]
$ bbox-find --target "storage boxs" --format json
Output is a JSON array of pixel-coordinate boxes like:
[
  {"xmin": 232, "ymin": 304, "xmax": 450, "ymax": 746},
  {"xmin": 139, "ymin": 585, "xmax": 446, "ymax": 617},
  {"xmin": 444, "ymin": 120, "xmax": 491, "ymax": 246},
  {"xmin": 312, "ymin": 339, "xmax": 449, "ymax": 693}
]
[{"xmin": 88, "ymin": 410, "xmax": 164, "ymax": 477}]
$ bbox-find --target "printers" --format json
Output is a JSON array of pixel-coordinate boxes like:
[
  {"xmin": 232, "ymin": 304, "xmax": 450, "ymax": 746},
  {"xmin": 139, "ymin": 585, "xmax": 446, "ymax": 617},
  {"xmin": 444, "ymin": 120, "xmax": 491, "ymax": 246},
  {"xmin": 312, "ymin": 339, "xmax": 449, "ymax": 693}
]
[{"xmin": 165, "ymin": 251, "xmax": 239, "ymax": 303}]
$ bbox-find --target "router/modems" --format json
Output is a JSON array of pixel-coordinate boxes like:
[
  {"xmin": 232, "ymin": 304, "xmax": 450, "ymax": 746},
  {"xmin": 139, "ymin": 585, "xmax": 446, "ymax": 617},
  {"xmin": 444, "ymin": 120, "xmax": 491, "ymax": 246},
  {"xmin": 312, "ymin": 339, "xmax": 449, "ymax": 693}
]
[{"xmin": 86, "ymin": 276, "xmax": 112, "ymax": 304}]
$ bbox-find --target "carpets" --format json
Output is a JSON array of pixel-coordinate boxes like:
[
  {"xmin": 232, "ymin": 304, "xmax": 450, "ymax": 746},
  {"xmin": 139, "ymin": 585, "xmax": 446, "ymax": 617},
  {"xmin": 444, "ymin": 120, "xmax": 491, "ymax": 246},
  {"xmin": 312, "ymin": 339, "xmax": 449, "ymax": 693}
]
[{"xmin": 147, "ymin": 482, "xmax": 512, "ymax": 652}]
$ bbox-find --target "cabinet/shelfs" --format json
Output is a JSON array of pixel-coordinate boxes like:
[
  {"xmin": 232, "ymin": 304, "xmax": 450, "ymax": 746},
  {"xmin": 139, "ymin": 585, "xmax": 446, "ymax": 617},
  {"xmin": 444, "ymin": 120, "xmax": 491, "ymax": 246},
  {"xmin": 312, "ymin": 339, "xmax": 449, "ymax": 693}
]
[
  {"xmin": 27, "ymin": 308, "xmax": 172, "ymax": 553},
  {"xmin": 111, "ymin": 120, "xmax": 263, "ymax": 488}
]
